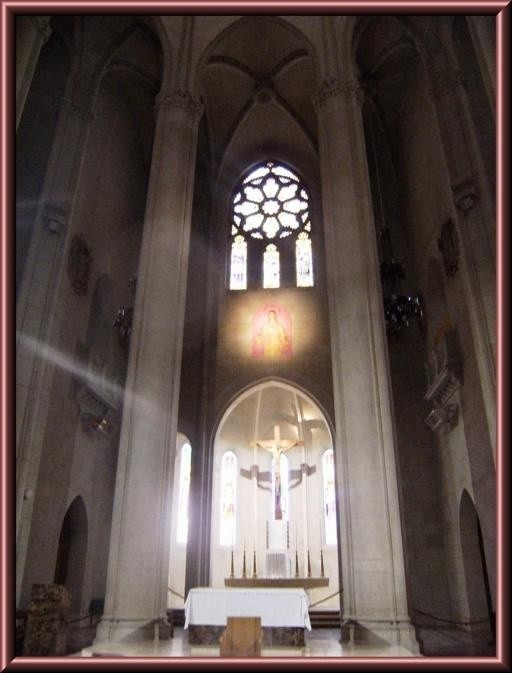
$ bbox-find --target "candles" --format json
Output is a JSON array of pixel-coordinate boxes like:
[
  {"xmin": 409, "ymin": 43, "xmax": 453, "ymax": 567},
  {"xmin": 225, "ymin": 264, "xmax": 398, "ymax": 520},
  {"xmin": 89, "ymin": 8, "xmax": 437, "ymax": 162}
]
[{"xmin": 230, "ymin": 549, "xmax": 325, "ymax": 574}]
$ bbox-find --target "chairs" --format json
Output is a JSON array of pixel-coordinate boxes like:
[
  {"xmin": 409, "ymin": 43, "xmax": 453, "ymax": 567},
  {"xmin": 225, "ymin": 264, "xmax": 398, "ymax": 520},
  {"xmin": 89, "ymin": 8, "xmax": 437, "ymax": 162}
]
[{"xmin": 218, "ymin": 615, "xmax": 264, "ymax": 658}]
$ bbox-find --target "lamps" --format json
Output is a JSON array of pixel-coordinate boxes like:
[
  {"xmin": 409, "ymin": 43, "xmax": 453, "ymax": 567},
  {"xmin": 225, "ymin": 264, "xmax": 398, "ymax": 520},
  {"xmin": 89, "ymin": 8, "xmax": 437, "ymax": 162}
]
[
  {"xmin": 423, "ymin": 400, "xmax": 459, "ymax": 430},
  {"xmin": 111, "ymin": 276, "xmax": 138, "ymax": 351},
  {"xmin": 83, "ymin": 405, "xmax": 119, "ymax": 439},
  {"xmin": 364, "ymin": 116, "xmax": 423, "ymax": 346}
]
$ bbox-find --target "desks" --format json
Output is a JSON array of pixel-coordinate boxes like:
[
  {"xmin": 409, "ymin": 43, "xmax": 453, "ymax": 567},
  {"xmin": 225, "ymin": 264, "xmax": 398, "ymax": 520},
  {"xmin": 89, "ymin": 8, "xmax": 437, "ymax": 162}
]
[{"xmin": 183, "ymin": 586, "xmax": 312, "ymax": 649}]
